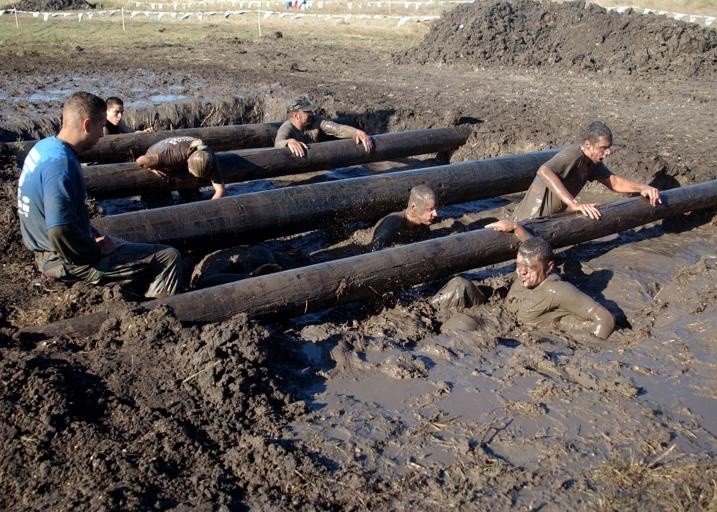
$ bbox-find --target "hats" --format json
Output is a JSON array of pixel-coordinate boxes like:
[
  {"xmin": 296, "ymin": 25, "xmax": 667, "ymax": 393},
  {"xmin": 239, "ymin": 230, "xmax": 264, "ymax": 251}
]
[{"xmin": 286, "ymin": 96, "xmax": 317, "ymax": 113}]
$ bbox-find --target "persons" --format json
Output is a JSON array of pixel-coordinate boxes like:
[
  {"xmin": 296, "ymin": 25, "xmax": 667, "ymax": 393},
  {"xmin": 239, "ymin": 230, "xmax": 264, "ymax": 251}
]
[
  {"xmin": 508, "ymin": 117, "xmax": 664, "ymax": 217},
  {"xmin": 271, "ymin": 97, "xmax": 379, "ymax": 158},
  {"xmin": 427, "ymin": 221, "xmax": 616, "ymax": 342},
  {"xmin": 135, "ymin": 133, "xmax": 226, "ymax": 201},
  {"xmin": 367, "ymin": 182, "xmax": 440, "ymax": 250},
  {"xmin": 100, "ymin": 95, "xmax": 156, "ymax": 137},
  {"xmin": 16, "ymin": 86, "xmax": 188, "ymax": 303}
]
